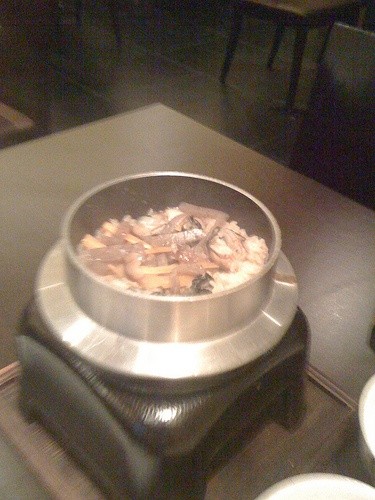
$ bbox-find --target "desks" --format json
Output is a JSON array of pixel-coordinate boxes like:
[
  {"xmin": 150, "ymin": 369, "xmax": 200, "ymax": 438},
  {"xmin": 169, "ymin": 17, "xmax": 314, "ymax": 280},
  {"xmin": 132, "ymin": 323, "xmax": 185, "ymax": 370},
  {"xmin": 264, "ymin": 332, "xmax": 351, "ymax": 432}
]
[{"xmin": 0, "ymin": 103, "xmax": 374, "ymax": 499}]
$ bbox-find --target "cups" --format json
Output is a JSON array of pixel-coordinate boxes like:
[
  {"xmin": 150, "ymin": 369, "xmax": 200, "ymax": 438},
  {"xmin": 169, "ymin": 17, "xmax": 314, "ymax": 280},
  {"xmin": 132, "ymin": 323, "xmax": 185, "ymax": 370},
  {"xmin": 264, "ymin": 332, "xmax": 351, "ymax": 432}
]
[
  {"xmin": 356, "ymin": 375, "xmax": 375, "ymax": 473},
  {"xmin": 256, "ymin": 472, "xmax": 375, "ymax": 500}
]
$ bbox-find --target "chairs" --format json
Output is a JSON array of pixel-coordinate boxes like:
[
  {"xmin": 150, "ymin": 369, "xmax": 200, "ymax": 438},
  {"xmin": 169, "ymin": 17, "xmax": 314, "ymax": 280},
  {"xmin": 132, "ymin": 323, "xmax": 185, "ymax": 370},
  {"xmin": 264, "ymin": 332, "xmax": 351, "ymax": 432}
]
[{"xmin": 219, "ymin": 0, "xmax": 340, "ymax": 110}]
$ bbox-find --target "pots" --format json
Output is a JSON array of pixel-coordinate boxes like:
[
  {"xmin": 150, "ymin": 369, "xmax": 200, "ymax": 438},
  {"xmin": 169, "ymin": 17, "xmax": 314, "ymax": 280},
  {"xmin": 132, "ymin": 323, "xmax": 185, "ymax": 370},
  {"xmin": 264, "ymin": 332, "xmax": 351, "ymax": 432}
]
[{"xmin": 35, "ymin": 170, "xmax": 301, "ymax": 383}]
